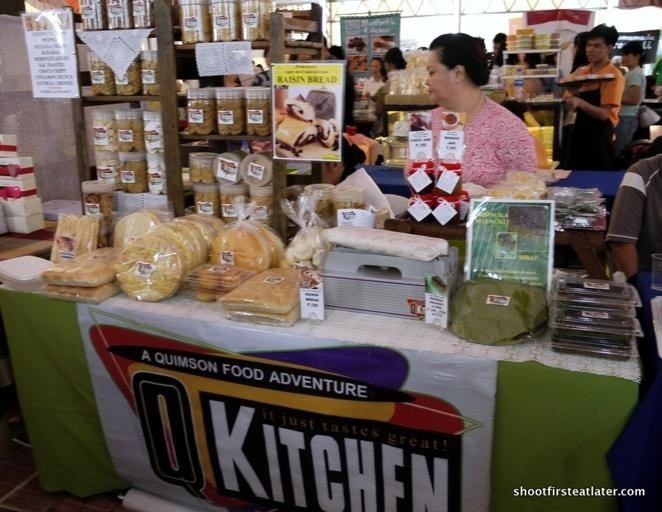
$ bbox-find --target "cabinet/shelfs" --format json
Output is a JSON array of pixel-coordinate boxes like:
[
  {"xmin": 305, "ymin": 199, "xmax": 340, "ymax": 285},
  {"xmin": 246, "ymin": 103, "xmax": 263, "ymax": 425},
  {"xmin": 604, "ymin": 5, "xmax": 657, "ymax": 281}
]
[
  {"xmin": 499, "ymin": 48, "xmax": 563, "ymax": 92},
  {"xmin": 166, "ymin": 35, "xmax": 325, "ymax": 245},
  {"xmin": 65, "ymin": 19, "xmax": 187, "ymax": 219},
  {"xmin": 375, "ymin": 93, "xmax": 565, "ymax": 178}
]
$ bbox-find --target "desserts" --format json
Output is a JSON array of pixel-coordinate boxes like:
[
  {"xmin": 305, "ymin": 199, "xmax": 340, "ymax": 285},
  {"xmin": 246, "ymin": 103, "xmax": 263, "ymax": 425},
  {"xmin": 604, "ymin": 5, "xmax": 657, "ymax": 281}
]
[{"xmin": 283, "ymin": 225, "xmax": 330, "ymax": 268}]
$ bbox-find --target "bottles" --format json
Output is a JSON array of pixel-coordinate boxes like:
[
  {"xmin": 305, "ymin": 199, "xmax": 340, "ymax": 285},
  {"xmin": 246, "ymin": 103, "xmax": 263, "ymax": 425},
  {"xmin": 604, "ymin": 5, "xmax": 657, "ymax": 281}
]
[
  {"xmin": 488, "ymin": 68, "xmax": 497, "ymax": 83},
  {"xmin": 504, "ymin": 64, "xmax": 515, "ymax": 74},
  {"xmin": 513, "ymin": 66, "xmax": 525, "ymax": 101}
]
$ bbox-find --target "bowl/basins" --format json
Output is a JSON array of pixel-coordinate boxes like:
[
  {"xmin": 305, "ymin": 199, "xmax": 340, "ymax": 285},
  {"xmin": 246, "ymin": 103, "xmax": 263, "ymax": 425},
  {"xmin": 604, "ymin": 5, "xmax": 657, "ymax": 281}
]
[
  {"xmin": 525, "ymin": 64, "xmax": 557, "ymax": 74},
  {"xmin": 505, "ymin": 27, "xmax": 561, "ymax": 49},
  {"xmin": 559, "ymin": 73, "xmax": 615, "ymax": 82}
]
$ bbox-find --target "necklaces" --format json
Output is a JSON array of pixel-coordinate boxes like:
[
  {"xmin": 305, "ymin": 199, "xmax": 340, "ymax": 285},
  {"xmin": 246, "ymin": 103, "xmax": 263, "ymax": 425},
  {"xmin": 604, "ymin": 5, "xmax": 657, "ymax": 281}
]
[{"xmin": 466, "ymin": 93, "xmax": 480, "ymax": 122}]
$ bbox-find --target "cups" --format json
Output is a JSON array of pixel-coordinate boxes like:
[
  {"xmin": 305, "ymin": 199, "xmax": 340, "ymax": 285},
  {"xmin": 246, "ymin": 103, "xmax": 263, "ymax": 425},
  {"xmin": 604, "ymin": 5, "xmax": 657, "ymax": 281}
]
[
  {"xmin": 440, "ymin": 112, "xmax": 463, "ymax": 130},
  {"xmin": 650, "ymin": 253, "xmax": 662, "ymax": 292}
]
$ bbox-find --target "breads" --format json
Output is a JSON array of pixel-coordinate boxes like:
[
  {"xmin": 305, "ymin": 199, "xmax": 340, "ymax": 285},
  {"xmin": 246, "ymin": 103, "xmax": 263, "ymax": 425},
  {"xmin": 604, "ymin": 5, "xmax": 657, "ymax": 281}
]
[
  {"xmin": 39, "ymin": 208, "xmax": 320, "ymax": 329},
  {"xmin": 275, "ymin": 101, "xmax": 339, "ymax": 150}
]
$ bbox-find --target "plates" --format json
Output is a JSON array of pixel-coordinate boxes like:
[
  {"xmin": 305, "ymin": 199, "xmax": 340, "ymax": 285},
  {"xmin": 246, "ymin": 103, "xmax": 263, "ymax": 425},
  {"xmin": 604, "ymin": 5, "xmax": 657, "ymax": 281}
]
[
  {"xmin": 371, "ymin": 38, "xmax": 396, "ymax": 52},
  {"xmin": 346, "ymin": 38, "xmax": 369, "ymax": 53}
]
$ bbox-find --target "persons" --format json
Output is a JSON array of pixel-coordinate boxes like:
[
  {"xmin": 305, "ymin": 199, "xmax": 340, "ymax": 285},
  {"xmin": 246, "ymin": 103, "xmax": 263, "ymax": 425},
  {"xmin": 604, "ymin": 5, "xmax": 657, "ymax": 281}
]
[
  {"xmin": 605, "ymin": 153, "xmax": 661, "ymax": 281},
  {"xmin": 405, "ymin": 33, "xmax": 537, "ymax": 198},
  {"xmin": 486, "ymin": 27, "xmax": 648, "ymax": 169},
  {"xmin": 290, "ymin": 34, "xmax": 406, "ymax": 141}
]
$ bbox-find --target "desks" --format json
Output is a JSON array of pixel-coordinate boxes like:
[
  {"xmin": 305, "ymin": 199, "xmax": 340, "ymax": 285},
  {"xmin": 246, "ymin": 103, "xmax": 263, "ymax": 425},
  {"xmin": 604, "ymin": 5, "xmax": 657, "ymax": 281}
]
[
  {"xmin": 0, "ymin": 253, "xmax": 645, "ymax": 512},
  {"xmin": 337, "ymin": 163, "xmax": 623, "ymax": 274},
  {"xmin": 2, "ymin": 217, "xmax": 63, "ymax": 431},
  {"xmin": 602, "ymin": 280, "xmax": 662, "ymax": 510}
]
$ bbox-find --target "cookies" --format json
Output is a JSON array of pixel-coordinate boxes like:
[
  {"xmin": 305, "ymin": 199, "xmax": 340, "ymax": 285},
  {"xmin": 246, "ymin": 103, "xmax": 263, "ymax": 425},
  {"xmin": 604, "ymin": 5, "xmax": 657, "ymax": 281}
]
[{"xmin": 73, "ymin": 0, "xmax": 277, "ymax": 228}]
